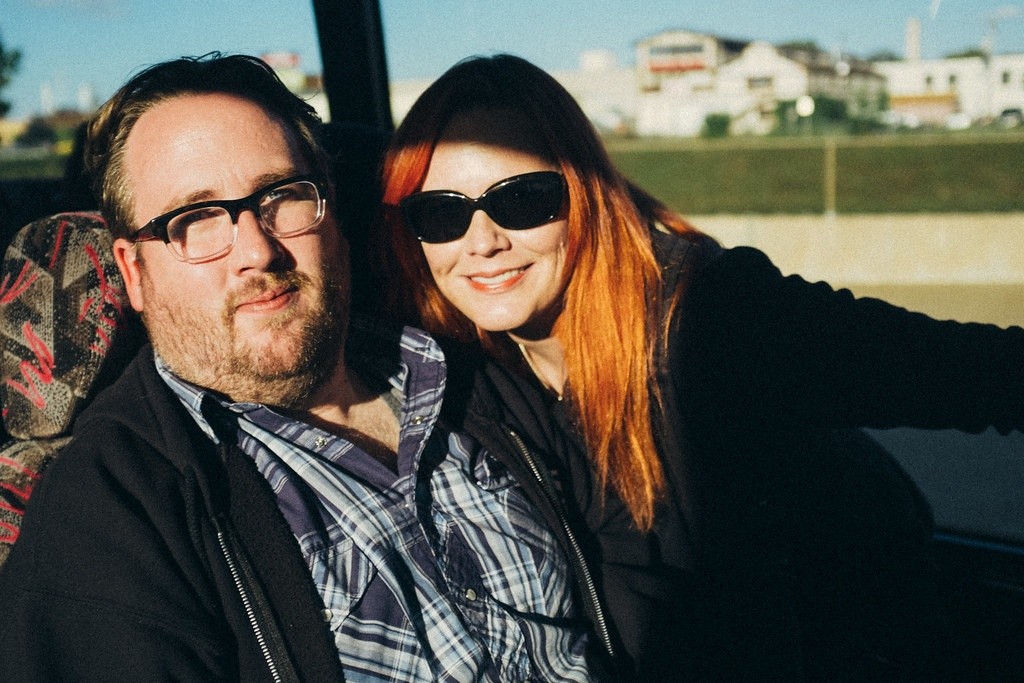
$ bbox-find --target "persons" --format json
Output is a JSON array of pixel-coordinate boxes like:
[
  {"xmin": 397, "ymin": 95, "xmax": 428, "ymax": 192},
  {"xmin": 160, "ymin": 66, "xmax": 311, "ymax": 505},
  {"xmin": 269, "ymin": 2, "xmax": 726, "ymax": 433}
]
[
  {"xmin": 0, "ymin": 56, "xmax": 762, "ymax": 683},
  {"xmin": 379, "ymin": 55, "xmax": 1024, "ymax": 683}
]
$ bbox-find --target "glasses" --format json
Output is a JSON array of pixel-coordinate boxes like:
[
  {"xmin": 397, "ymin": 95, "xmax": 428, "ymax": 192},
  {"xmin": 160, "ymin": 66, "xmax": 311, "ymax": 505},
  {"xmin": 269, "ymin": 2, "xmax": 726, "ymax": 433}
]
[
  {"xmin": 401, "ymin": 171, "xmax": 569, "ymax": 243},
  {"xmin": 125, "ymin": 171, "xmax": 326, "ymax": 265}
]
